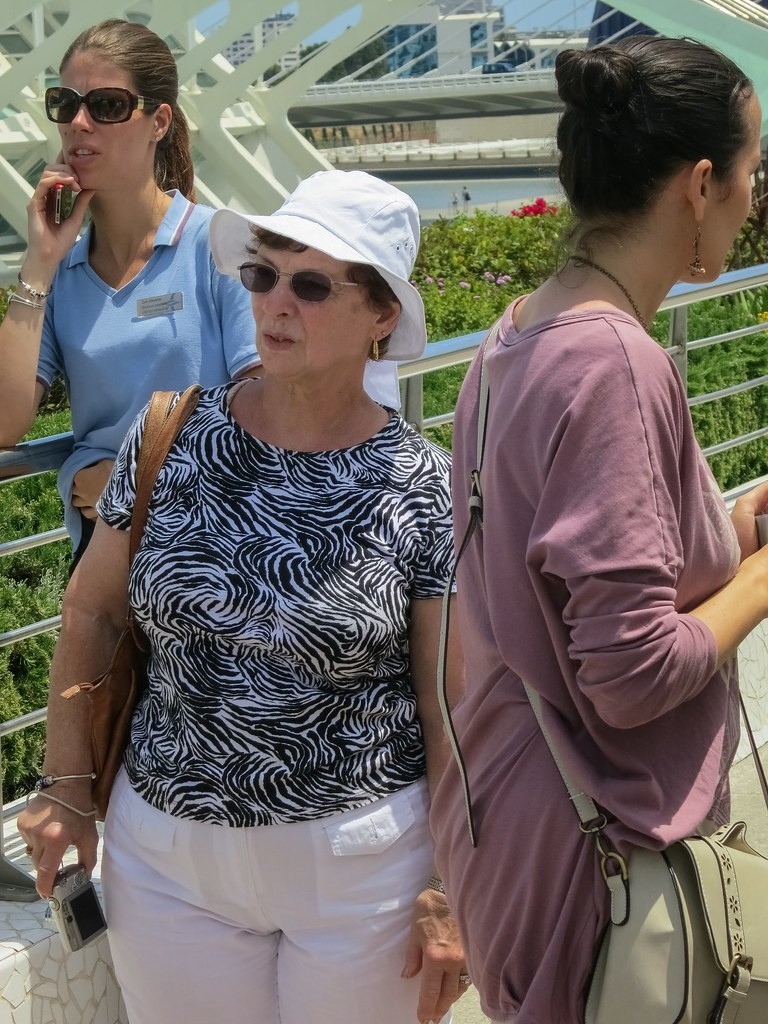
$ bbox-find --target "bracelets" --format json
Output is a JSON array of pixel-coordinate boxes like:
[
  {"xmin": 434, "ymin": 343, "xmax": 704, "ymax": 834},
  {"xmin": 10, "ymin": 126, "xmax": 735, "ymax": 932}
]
[
  {"xmin": 33, "ymin": 772, "xmax": 98, "ymax": 791},
  {"xmin": 17, "ymin": 272, "xmax": 53, "ymax": 298},
  {"xmin": 429, "ymin": 876, "xmax": 445, "ymax": 894},
  {"xmin": 7, "ymin": 292, "xmax": 44, "ymax": 309}
]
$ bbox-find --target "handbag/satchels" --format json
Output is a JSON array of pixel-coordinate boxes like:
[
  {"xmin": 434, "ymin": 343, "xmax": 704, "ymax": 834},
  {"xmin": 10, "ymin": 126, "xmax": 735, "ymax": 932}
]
[
  {"xmin": 585, "ymin": 821, "xmax": 768, "ymax": 1024},
  {"xmin": 59, "ymin": 383, "xmax": 206, "ymax": 822}
]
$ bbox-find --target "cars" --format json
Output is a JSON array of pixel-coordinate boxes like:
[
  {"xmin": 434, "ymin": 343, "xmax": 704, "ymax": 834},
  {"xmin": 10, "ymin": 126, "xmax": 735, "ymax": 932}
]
[{"xmin": 483, "ymin": 63, "xmax": 517, "ymax": 82}]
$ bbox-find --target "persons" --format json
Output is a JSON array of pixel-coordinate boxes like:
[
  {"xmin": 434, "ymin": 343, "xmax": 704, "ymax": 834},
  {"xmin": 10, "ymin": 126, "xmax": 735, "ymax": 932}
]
[
  {"xmin": 429, "ymin": 34, "xmax": 766, "ymax": 1023},
  {"xmin": 1, "ymin": 19, "xmax": 264, "ymax": 575},
  {"xmin": 16, "ymin": 170, "xmax": 465, "ymax": 1024}
]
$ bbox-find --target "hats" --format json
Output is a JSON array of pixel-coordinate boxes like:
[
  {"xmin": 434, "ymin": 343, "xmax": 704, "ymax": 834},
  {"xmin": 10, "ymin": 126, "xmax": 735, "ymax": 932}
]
[{"xmin": 208, "ymin": 171, "xmax": 427, "ymax": 362}]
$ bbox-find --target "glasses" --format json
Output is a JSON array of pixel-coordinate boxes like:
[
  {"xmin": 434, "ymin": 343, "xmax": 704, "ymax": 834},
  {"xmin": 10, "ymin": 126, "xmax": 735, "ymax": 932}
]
[
  {"xmin": 45, "ymin": 86, "xmax": 161, "ymax": 125},
  {"xmin": 237, "ymin": 261, "xmax": 369, "ymax": 303}
]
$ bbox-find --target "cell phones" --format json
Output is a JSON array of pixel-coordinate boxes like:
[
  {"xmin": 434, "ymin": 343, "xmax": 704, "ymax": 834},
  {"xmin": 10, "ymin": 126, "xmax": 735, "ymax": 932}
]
[{"xmin": 48, "ymin": 183, "xmax": 65, "ymax": 225}]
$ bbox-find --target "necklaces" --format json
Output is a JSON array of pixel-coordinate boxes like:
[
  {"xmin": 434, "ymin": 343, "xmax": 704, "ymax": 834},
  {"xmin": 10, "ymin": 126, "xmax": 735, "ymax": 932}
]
[{"xmin": 569, "ymin": 251, "xmax": 650, "ymax": 335}]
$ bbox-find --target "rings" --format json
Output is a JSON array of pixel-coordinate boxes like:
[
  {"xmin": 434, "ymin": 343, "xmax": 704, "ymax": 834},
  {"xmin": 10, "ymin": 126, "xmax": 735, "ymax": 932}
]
[
  {"xmin": 25, "ymin": 846, "xmax": 32, "ymax": 856},
  {"xmin": 459, "ymin": 974, "xmax": 470, "ymax": 985}
]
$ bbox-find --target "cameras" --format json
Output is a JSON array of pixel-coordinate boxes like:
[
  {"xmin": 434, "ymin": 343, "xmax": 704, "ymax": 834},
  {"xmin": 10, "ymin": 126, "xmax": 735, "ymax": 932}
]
[{"xmin": 47, "ymin": 864, "xmax": 109, "ymax": 953}]
